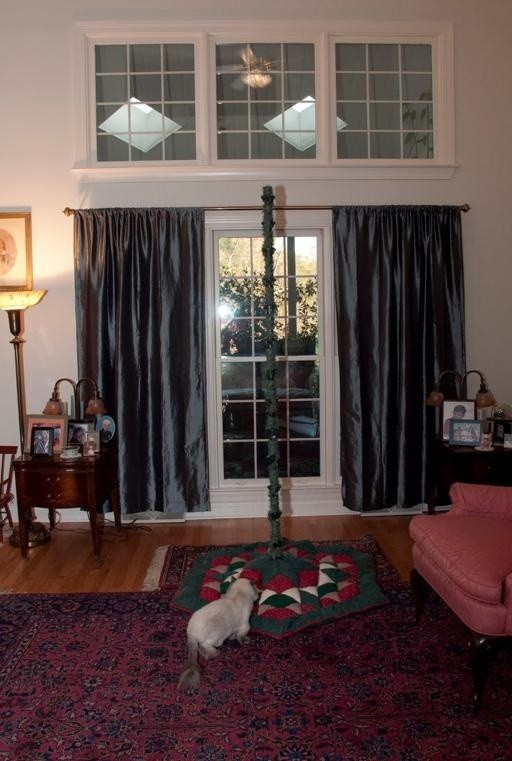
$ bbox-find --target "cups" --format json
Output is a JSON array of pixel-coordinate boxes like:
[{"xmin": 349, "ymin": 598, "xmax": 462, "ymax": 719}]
[{"xmin": 64, "ymin": 446, "xmax": 80, "ymax": 456}]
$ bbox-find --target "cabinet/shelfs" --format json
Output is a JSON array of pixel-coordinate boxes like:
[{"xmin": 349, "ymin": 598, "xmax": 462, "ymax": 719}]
[{"xmin": 13, "ymin": 451, "xmax": 122, "ymax": 567}]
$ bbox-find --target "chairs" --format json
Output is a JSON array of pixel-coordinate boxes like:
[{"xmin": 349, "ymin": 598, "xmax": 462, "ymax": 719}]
[{"xmin": 0, "ymin": 446, "xmax": 19, "ymax": 542}]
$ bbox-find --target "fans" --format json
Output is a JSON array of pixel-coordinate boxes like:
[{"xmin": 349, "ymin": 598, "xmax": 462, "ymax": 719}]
[{"xmin": 215, "ymin": 44, "xmax": 294, "ymax": 90}]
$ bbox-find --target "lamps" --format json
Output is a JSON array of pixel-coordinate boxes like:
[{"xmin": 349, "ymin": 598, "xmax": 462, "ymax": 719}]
[
  {"xmin": 0, "ymin": 288, "xmax": 50, "ymax": 549},
  {"xmin": 426, "ymin": 369, "xmax": 496, "ymax": 408},
  {"xmin": 263, "ymin": 96, "xmax": 349, "ymax": 153},
  {"xmin": 97, "ymin": 96, "xmax": 183, "ymax": 153},
  {"xmin": 242, "ymin": 63, "xmax": 272, "ymax": 88},
  {"xmin": 43, "ymin": 378, "xmax": 107, "ymax": 419}
]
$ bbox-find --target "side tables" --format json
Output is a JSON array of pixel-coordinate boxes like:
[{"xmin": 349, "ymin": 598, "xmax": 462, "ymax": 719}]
[{"xmin": 427, "ymin": 439, "xmax": 512, "ymax": 512}]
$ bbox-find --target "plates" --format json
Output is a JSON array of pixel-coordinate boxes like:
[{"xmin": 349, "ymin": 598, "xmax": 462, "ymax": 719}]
[{"xmin": 60, "ymin": 454, "xmax": 82, "ymax": 461}]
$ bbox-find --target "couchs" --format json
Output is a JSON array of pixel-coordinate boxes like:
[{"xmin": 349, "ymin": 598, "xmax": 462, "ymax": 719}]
[{"xmin": 408, "ymin": 482, "xmax": 512, "ymax": 719}]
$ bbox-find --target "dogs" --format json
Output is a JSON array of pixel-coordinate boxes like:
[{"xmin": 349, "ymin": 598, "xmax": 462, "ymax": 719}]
[{"xmin": 176, "ymin": 578, "xmax": 259, "ymax": 693}]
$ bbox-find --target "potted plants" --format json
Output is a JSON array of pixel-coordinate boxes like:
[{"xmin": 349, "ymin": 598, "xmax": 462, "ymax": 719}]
[
  {"xmin": 222, "ymin": 282, "xmax": 276, "ymax": 390},
  {"xmin": 276, "ymin": 281, "xmax": 317, "ymax": 386}
]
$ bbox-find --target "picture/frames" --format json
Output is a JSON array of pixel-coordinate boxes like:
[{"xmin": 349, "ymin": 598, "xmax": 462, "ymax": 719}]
[
  {"xmin": 0, "ymin": 212, "xmax": 33, "ymax": 291},
  {"xmin": 29, "ymin": 426, "xmax": 55, "ymax": 457},
  {"xmin": 450, "ymin": 418, "xmax": 483, "ymax": 446},
  {"xmin": 441, "ymin": 400, "xmax": 477, "ymax": 442},
  {"xmin": 96, "ymin": 414, "xmax": 116, "ymax": 445},
  {"xmin": 24, "ymin": 414, "xmax": 68, "ymax": 455},
  {"xmin": 67, "ymin": 417, "xmax": 96, "ymax": 447}
]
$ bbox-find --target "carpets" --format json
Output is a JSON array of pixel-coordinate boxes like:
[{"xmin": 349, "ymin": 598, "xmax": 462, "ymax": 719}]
[
  {"xmin": 142, "ymin": 536, "xmax": 400, "ymax": 590},
  {"xmin": 0, "ymin": 582, "xmax": 512, "ymax": 730}
]
